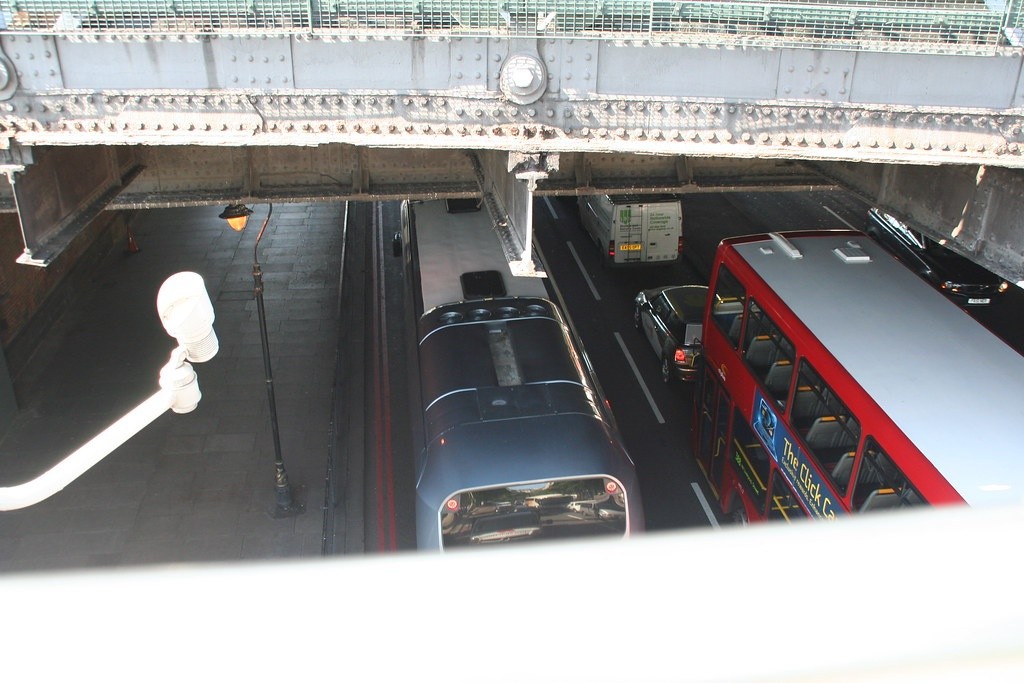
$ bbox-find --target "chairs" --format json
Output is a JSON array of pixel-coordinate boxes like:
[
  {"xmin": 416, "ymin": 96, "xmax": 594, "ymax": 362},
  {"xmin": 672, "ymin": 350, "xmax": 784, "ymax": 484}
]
[
  {"xmin": 780, "ymin": 338, "xmax": 795, "ymax": 362},
  {"xmin": 766, "ymin": 360, "xmax": 922, "ymax": 513},
  {"xmin": 758, "ymin": 315, "xmax": 778, "ymax": 335},
  {"xmin": 746, "ymin": 335, "xmax": 785, "ymax": 368},
  {"xmin": 728, "ymin": 313, "xmax": 760, "ymax": 349}
]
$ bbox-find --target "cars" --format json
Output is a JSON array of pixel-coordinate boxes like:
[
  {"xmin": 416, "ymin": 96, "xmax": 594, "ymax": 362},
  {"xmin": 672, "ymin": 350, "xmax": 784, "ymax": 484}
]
[{"xmin": 864, "ymin": 209, "xmax": 1009, "ymax": 314}]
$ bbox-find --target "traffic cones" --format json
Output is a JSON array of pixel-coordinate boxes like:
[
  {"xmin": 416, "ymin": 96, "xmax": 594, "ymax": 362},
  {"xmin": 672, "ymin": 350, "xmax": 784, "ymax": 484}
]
[{"xmin": 126, "ymin": 227, "xmax": 139, "ymax": 253}]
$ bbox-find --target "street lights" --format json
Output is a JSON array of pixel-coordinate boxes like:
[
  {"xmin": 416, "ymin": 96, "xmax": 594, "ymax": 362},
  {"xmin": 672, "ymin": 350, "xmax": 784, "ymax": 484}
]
[{"xmin": 217, "ymin": 201, "xmax": 308, "ymax": 516}]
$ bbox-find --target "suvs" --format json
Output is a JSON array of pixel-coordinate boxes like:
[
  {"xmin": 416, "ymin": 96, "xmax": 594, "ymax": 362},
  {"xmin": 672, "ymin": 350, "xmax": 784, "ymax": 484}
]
[{"xmin": 633, "ymin": 285, "xmax": 724, "ymax": 386}]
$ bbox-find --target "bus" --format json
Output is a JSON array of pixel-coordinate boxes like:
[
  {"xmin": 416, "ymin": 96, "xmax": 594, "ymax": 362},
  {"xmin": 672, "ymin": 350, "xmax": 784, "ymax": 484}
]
[
  {"xmin": 692, "ymin": 226, "xmax": 1024, "ymax": 534},
  {"xmin": 392, "ymin": 195, "xmax": 648, "ymax": 553}
]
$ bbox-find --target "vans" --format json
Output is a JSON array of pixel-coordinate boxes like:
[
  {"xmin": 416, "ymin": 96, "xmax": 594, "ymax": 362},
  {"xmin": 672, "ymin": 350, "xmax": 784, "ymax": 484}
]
[{"xmin": 578, "ymin": 194, "xmax": 687, "ymax": 268}]
